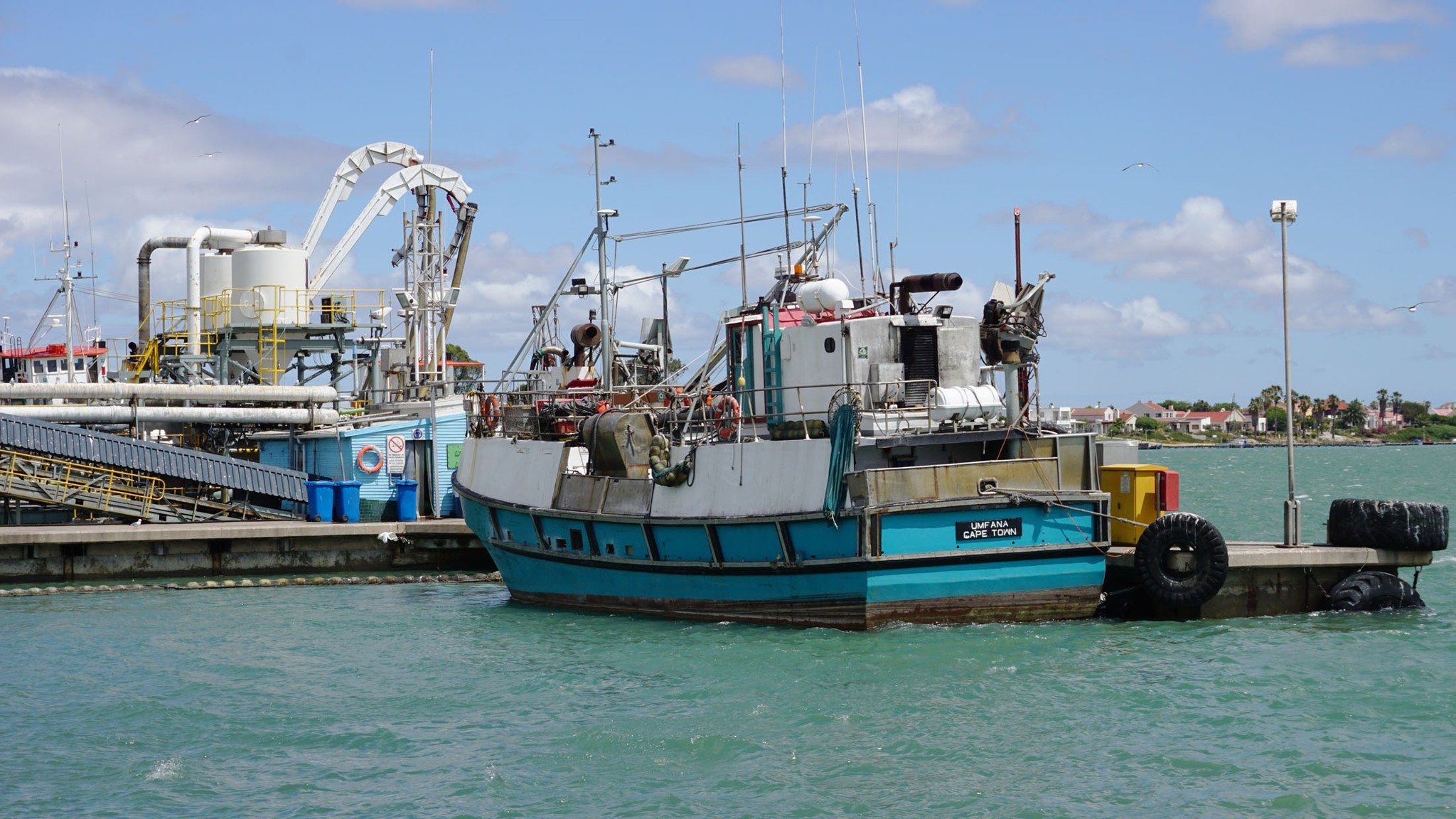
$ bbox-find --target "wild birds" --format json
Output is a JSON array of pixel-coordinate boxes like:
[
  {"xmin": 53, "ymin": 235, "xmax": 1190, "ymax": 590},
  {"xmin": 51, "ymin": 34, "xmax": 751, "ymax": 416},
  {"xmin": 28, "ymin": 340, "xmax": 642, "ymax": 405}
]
[
  {"xmin": 1387, "ymin": 300, "xmax": 1438, "ymax": 313},
  {"xmin": 198, "ymin": 151, "xmax": 223, "ymax": 158},
  {"xmin": 1121, "ymin": 163, "xmax": 1160, "ymax": 173},
  {"xmin": 184, "ymin": 114, "xmax": 210, "ymax": 126}
]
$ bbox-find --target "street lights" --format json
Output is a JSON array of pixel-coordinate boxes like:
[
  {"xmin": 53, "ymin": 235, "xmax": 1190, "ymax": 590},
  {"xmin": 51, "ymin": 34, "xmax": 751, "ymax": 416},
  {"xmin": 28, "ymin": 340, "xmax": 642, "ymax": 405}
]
[{"xmin": 1268, "ymin": 195, "xmax": 1306, "ymax": 546}]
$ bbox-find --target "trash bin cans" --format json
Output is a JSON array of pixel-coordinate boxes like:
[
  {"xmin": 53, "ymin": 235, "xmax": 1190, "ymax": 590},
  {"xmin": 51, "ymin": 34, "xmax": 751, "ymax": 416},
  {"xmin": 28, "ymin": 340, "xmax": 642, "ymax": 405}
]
[
  {"xmin": 1098, "ymin": 464, "xmax": 1168, "ymax": 545},
  {"xmin": 453, "ymin": 490, "xmax": 463, "ymax": 518},
  {"xmin": 303, "ymin": 481, "xmax": 337, "ymax": 522},
  {"xmin": 395, "ymin": 479, "xmax": 418, "ymax": 522},
  {"xmin": 333, "ymin": 481, "xmax": 363, "ymax": 523}
]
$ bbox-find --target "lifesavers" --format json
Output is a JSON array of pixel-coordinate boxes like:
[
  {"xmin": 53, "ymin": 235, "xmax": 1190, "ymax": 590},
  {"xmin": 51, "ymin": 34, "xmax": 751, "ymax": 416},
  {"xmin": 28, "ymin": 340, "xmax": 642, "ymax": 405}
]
[
  {"xmin": 1327, "ymin": 498, "xmax": 1450, "ymax": 551},
  {"xmin": 714, "ymin": 395, "xmax": 739, "ymax": 438},
  {"xmin": 356, "ymin": 445, "xmax": 384, "ymax": 474},
  {"xmin": 1133, "ymin": 512, "xmax": 1229, "ymax": 608},
  {"xmin": 485, "ymin": 395, "xmax": 500, "ymax": 427},
  {"xmin": 1322, "ymin": 570, "xmax": 1426, "ymax": 611},
  {"xmin": 663, "ymin": 389, "xmax": 692, "ymax": 407}
]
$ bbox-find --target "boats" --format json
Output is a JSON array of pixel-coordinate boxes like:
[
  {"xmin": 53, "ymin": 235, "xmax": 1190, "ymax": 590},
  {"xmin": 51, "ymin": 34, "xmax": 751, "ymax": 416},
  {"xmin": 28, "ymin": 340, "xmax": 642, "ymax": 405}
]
[{"xmin": 450, "ymin": 40, "xmax": 1118, "ymax": 630}]
